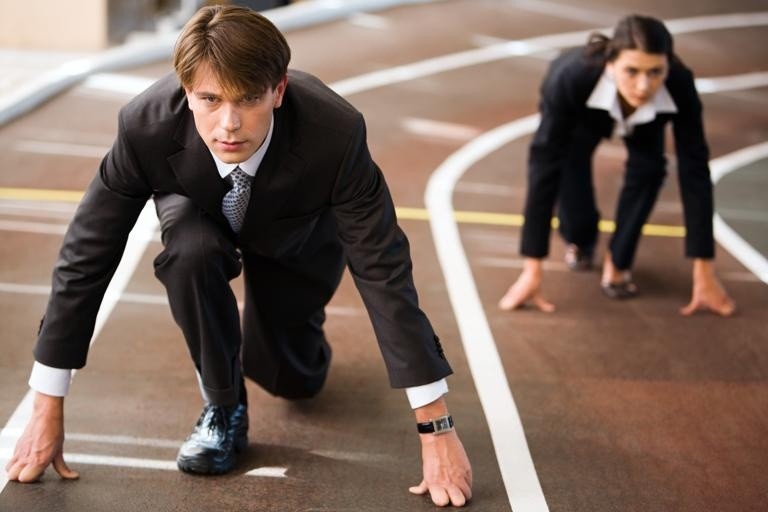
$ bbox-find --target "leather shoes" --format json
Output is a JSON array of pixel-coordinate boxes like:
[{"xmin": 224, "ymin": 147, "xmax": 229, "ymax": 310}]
[
  {"xmin": 602, "ymin": 257, "xmax": 638, "ymax": 302},
  {"xmin": 562, "ymin": 245, "xmax": 597, "ymax": 273}
]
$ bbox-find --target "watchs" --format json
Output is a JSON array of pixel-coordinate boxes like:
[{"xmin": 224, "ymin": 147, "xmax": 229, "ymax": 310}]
[{"xmin": 415, "ymin": 413, "xmax": 461, "ymax": 437}]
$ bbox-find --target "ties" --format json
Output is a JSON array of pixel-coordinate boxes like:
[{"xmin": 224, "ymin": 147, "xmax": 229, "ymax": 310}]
[{"xmin": 220, "ymin": 168, "xmax": 253, "ymax": 231}]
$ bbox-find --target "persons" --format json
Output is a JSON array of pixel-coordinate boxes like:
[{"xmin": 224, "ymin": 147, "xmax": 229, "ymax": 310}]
[
  {"xmin": 499, "ymin": 13, "xmax": 738, "ymax": 320},
  {"xmin": 4, "ymin": 4, "xmax": 475, "ymax": 507}
]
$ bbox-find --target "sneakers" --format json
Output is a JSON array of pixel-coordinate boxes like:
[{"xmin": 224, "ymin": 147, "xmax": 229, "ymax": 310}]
[{"xmin": 176, "ymin": 400, "xmax": 251, "ymax": 479}]
